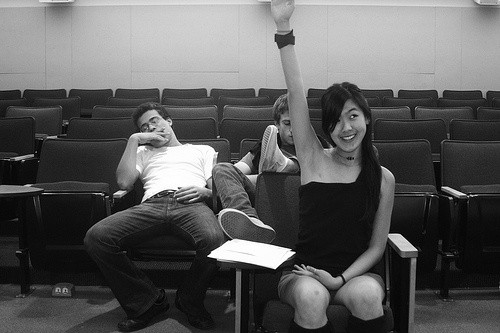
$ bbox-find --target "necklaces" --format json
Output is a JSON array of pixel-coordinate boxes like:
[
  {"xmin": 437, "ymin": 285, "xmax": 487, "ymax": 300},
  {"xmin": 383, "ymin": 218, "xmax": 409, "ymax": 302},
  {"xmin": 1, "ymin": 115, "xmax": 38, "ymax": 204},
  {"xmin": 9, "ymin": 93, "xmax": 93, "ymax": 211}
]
[{"xmin": 334, "ymin": 147, "xmax": 362, "ymax": 160}]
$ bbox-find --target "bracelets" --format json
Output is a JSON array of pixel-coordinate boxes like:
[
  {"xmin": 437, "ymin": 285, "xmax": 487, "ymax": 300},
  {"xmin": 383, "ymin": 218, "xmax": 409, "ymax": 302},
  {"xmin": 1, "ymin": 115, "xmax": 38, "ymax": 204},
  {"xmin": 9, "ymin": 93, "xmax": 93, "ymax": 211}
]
[
  {"xmin": 275, "ymin": 29, "xmax": 295, "ymax": 49},
  {"xmin": 336, "ymin": 272, "xmax": 346, "ymax": 287}
]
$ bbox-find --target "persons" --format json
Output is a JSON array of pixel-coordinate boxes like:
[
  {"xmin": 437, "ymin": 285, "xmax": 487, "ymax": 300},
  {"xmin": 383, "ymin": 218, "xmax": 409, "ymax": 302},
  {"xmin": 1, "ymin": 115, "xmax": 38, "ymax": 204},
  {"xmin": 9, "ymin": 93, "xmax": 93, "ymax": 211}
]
[
  {"xmin": 269, "ymin": 0, "xmax": 395, "ymax": 333},
  {"xmin": 84, "ymin": 102, "xmax": 223, "ymax": 332},
  {"xmin": 212, "ymin": 92, "xmax": 334, "ymax": 245}
]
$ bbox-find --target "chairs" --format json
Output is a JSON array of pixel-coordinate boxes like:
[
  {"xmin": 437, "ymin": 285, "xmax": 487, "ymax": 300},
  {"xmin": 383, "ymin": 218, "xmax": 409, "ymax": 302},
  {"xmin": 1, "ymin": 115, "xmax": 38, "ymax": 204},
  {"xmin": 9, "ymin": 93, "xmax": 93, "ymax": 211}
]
[{"xmin": 0, "ymin": 88, "xmax": 500, "ymax": 333}]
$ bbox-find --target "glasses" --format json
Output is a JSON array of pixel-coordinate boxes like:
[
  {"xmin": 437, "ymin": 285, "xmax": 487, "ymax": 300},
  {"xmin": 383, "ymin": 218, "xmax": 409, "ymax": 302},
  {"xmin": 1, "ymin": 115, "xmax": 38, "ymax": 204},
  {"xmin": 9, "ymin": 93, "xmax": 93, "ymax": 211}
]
[{"xmin": 140, "ymin": 118, "xmax": 160, "ymax": 133}]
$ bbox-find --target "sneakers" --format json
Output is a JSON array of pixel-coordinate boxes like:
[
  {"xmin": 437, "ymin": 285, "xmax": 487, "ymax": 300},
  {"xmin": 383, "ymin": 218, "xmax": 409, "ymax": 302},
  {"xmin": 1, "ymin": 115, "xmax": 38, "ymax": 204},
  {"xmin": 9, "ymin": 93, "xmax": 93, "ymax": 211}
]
[
  {"xmin": 118, "ymin": 290, "xmax": 169, "ymax": 331},
  {"xmin": 218, "ymin": 208, "xmax": 276, "ymax": 243},
  {"xmin": 259, "ymin": 125, "xmax": 289, "ymax": 175},
  {"xmin": 175, "ymin": 290, "xmax": 215, "ymax": 331}
]
